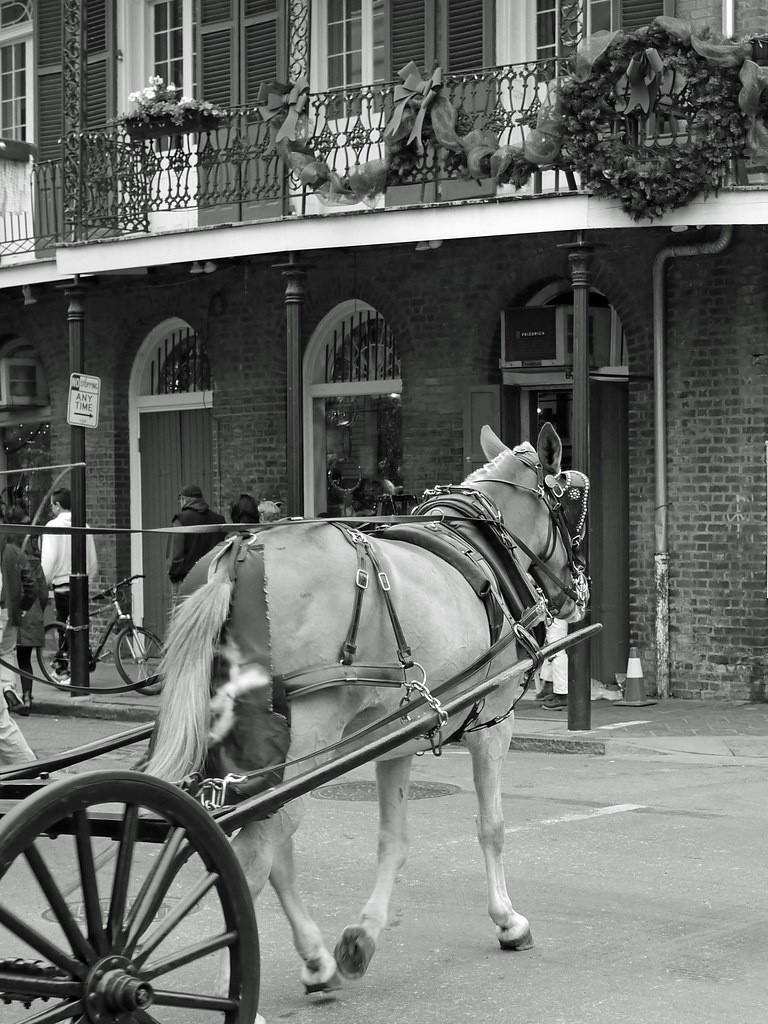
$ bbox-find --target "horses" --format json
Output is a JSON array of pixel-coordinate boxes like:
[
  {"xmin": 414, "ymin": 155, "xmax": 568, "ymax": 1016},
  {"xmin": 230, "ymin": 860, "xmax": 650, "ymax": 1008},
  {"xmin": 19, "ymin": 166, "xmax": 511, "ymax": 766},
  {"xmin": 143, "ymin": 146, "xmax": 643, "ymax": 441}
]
[{"xmin": 138, "ymin": 421, "xmax": 590, "ymax": 1023}]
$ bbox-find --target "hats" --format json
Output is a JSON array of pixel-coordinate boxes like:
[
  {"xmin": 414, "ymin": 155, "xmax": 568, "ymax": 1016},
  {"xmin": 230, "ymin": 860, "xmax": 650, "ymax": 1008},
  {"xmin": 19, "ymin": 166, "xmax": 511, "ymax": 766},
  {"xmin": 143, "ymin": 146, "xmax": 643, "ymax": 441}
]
[{"xmin": 176, "ymin": 485, "xmax": 203, "ymax": 498}]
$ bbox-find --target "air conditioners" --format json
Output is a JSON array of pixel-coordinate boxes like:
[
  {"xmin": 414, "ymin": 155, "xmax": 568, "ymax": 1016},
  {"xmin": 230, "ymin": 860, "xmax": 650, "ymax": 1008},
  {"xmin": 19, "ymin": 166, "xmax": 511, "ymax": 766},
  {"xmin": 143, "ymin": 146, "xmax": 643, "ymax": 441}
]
[
  {"xmin": 498, "ymin": 305, "xmax": 611, "ymax": 368},
  {"xmin": 0, "ymin": 357, "xmax": 50, "ymax": 406}
]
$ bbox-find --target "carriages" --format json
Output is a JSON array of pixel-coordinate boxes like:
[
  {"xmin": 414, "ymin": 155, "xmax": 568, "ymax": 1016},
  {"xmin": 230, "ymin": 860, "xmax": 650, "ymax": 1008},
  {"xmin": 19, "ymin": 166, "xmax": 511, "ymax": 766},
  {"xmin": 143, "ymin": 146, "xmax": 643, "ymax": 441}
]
[{"xmin": 0, "ymin": 420, "xmax": 607, "ymax": 1023}]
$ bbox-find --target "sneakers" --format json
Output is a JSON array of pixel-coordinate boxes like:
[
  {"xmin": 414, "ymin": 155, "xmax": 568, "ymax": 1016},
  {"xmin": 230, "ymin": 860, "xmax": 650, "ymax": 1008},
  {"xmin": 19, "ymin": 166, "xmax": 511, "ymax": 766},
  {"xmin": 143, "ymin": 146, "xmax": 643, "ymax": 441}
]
[{"xmin": 3, "ymin": 686, "xmax": 25, "ymax": 713}]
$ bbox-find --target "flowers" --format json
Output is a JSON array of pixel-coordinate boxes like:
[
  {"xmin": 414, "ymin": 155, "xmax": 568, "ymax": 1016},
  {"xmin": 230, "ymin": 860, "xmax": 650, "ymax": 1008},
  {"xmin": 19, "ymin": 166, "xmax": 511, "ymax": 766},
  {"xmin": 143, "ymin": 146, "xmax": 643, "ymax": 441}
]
[{"xmin": 102, "ymin": 74, "xmax": 229, "ymax": 126}]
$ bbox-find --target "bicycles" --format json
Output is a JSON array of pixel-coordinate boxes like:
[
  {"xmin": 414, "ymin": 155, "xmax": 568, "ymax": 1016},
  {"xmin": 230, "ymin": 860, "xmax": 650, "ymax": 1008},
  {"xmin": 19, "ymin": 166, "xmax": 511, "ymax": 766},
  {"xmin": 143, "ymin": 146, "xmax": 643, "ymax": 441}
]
[{"xmin": 35, "ymin": 573, "xmax": 170, "ymax": 696}]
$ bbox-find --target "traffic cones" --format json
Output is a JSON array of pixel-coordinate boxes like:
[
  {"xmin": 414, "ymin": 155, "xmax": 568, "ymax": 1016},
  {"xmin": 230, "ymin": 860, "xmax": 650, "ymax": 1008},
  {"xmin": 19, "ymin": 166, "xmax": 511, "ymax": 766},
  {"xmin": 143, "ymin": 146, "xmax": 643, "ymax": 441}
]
[{"xmin": 610, "ymin": 646, "xmax": 659, "ymax": 707}]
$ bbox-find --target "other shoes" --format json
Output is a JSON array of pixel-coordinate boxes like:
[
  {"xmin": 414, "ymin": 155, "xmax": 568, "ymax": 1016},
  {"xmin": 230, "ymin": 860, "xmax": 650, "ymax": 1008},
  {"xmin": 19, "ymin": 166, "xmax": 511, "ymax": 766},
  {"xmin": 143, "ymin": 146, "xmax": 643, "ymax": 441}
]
[
  {"xmin": 543, "ymin": 695, "xmax": 568, "ymax": 711},
  {"xmin": 536, "ymin": 681, "xmax": 553, "ymax": 699},
  {"xmin": 22, "ymin": 694, "xmax": 31, "ymax": 717},
  {"xmin": 51, "ymin": 672, "xmax": 70, "ymax": 683}
]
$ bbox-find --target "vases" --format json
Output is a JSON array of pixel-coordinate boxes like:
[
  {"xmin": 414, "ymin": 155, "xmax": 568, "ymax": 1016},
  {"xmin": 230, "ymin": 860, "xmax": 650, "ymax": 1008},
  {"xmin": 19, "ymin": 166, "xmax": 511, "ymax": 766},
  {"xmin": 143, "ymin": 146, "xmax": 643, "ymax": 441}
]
[{"xmin": 126, "ymin": 114, "xmax": 219, "ymax": 140}]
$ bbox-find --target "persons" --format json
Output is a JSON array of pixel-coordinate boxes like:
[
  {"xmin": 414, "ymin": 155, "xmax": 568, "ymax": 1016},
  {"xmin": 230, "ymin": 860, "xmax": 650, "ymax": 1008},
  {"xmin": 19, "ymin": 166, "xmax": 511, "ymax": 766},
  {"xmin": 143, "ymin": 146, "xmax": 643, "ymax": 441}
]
[
  {"xmin": 40, "ymin": 488, "xmax": 98, "ymax": 686},
  {"xmin": 232, "ymin": 494, "xmax": 260, "ymax": 532},
  {"xmin": 165, "ymin": 484, "xmax": 228, "ymax": 591},
  {"xmin": 0, "ymin": 684, "xmax": 37, "ymax": 766},
  {"xmin": 257, "ymin": 500, "xmax": 281, "ymax": 523},
  {"xmin": 0, "ymin": 521, "xmax": 49, "ymax": 715}
]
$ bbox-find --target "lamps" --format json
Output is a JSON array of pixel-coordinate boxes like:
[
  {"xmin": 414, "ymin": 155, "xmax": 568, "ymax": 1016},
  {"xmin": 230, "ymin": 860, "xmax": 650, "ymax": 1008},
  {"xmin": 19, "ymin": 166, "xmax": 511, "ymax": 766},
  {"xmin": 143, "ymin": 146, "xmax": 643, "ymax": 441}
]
[
  {"xmin": 415, "ymin": 240, "xmax": 443, "ymax": 251},
  {"xmin": 22, "ymin": 285, "xmax": 37, "ymax": 304},
  {"xmin": 671, "ymin": 225, "xmax": 709, "ymax": 232},
  {"xmin": 189, "ymin": 258, "xmax": 218, "ymax": 273}
]
